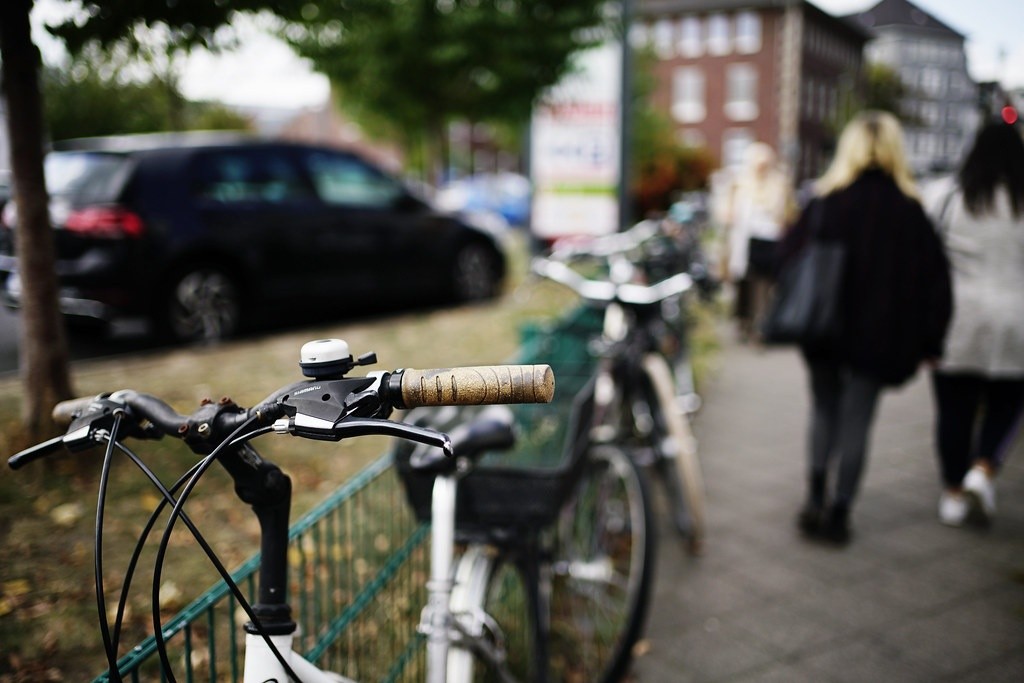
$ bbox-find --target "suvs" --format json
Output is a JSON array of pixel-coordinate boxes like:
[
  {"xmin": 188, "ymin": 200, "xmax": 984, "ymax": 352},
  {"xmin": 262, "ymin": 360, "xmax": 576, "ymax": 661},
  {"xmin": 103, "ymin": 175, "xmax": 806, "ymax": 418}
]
[{"xmin": 1, "ymin": 130, "xmax": 508, "ymax": 360}]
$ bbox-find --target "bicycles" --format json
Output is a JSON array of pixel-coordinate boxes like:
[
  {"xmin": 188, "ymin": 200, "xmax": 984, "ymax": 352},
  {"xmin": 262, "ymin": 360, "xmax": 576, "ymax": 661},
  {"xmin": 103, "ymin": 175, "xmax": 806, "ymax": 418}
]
[{"xmin": 6, "ymin": 256, "xmax": 710, "ymax": 683}]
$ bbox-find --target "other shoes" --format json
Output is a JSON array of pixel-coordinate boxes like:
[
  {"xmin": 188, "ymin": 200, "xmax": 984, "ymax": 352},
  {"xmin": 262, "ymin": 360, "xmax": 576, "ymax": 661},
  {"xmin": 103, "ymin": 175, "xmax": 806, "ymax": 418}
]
[{"xmin": 801, "ymin": 508, "xmax": 851, "ymax": 545}]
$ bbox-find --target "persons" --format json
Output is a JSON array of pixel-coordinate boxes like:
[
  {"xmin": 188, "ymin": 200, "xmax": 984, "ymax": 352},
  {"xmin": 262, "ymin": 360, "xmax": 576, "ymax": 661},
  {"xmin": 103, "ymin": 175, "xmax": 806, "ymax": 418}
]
[
  {"xmin": 710, "ymin": 140, "xmax": 796, "ymax": 345},
  {"xmin": 773, "ymin": 110, "xmax": 955, "ymax": 548},
  {"xmin": 922, "ymin": 116, "xmax": 1023, "ymax": 522}
]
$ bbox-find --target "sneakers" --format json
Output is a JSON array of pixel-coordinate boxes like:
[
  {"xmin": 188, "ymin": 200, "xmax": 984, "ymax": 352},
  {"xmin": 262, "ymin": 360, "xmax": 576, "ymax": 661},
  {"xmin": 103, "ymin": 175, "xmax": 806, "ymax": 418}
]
[
  {"xmin": 965, "ymin": 470, "xmax": 999, "ymax": 525},
  {"xmin": 939, "ymin": 494, "xmax": 973, "ymax": 526}
]
